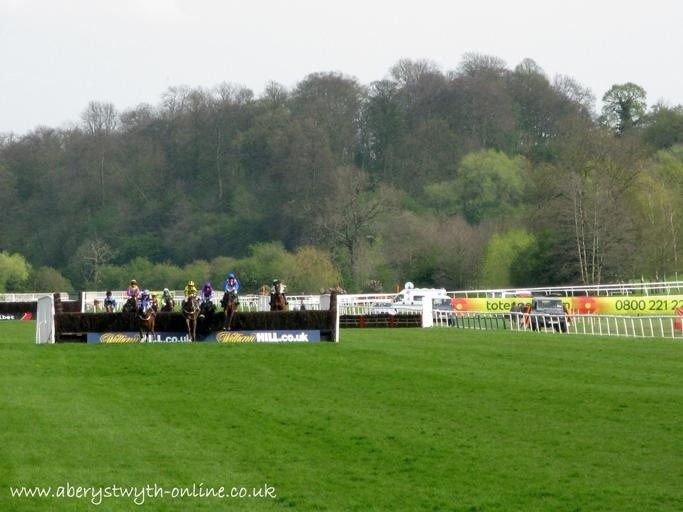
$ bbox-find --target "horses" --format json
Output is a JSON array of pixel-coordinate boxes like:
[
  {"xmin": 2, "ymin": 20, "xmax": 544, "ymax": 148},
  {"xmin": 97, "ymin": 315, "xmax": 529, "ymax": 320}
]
[
  {"xmin": 105, "ymin": 301, "xmax": 114, "ymax": 313},
  {"xmin": 223, "ymin": 291, "xmax": 237, "ymax": 331},
  {"xmin": 123, "ymin": 299, "xmax": 158, "ymax": 344},
  {"xmin": 181, "ymin": 297, "xmax": 217, "ymax": 341},
  {"xmin": 271, "ymin": 296, "xmax": 286, "ymax": 312},
  {"xmin": 161, "ymin": 299, "xmax": 174, "ymax": 312}
]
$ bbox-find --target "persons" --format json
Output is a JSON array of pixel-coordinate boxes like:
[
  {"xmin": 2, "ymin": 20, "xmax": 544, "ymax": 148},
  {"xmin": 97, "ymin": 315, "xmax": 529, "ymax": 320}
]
[
  {"xmin": 92, "ymin": 299, "xmax": 102, "ymax": 312},
  {"xmin": 124, "ymin": 279, "xmax": 139, "ymax": 312},
  {"xmin": 220, "ymin": 273, "xmax": 240, "ymax": 307},
  {"xmin": 135, "ymin": 289, "xmax": 152, "ymax": 321},
  {"xmin": 159, "ymin": 288, "xmax": 174, "ymax": 312},
  {"xmin": 508, "ymin": 301, "xmax": 518, "ymax": 327},
  {"xmin": 149, "ymin": 290, "xmax": 160, "ymax": 310},
  {"xmin": 270, "ymin": 278, "xmax": 289, "ymax": 306},
  {"xmin": 200, "ymin": 281, "xmax": 214, "ymax": 307},
  {"xmin": 102, "ymin": 291, "xmax": 115, "ymax": 309},
  {"xmin": 182, "ymin": 280, "xmax": 205, "ymax": 319}
]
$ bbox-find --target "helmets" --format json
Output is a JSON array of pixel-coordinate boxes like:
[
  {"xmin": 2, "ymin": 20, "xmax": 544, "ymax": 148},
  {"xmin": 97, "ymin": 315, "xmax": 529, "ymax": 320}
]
[
  {"xmin": 164, "ymin": 288, "xmax": 169, "ymax": 293},
  {"xmin": 273, "ymin": 279, "xmax": 280, "ymax": 285},
  {"xmin": 106, "ymin": 291, "xmax": 112, "ymax": 296},
  {"xmin": 227, "ymin": 273, "xmax": 234, "ymax": 278},
  {"xmin": 205, "ymin": 282, "xmax": 210, "ymax": 288},
  {"xmin": 131, "ymin": 280, "xmax": 136, "ymax": 283},
  {"xmin": 188, "ymin": 280, "xmax": 194, "ymax": 286},
  {"xmin": 144, "ymin": 290, "xmax": 149, "ymax": 296}
]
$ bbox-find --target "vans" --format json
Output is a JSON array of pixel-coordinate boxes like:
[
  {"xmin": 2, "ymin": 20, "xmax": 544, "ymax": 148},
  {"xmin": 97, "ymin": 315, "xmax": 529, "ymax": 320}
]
[{"xmin": 390, "ymin": 286, "xmax": 448, "ymax": 309}]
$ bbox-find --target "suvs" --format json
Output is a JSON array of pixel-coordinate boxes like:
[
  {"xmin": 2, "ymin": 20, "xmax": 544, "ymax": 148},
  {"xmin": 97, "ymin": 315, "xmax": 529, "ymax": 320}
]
[
  {"xmin": 429, "ymin": 295, "xmax": 458, "ymax": 326},
  {"xmin": 369, "ymin": 298, "xmax": 398, "ymax": 317},
  {"xmin": 527, "ymin": 296, "xmax": 570, "ymax": 332}
]
